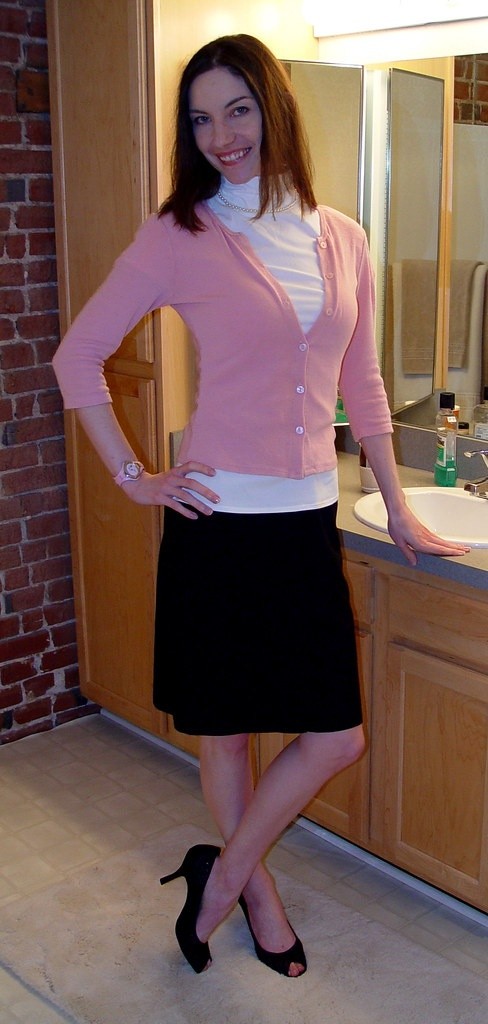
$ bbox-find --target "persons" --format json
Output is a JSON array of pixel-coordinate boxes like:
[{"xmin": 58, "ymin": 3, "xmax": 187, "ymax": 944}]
[{"xmin": 53, "ymin": 34, "xmax": 471, "ymax": 977}]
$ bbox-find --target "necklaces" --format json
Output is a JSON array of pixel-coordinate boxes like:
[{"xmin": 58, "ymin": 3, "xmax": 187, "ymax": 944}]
[{"xmin": 217, "ymin": 189, "xmax": 300, "ymax": 214}]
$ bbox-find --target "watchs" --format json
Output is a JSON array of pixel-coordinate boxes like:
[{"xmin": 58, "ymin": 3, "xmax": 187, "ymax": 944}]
[{"xmin": 112, "ymin": 460, "xmax": 144, "ymax": 487}]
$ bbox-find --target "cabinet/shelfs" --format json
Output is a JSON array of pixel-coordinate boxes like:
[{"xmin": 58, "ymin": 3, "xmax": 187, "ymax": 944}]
[
  {"xmin": 170, "ymin": 456, "xmax": 488, "ymax": 936},
  {"xmin": 47, "ymin": 0, "xmax": 317, "ymax": 769}
]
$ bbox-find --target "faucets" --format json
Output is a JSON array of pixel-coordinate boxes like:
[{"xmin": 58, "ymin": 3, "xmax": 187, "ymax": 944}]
[{"xmin": 463, "ymin": 448, "xmax": 488, "ymax": 493}]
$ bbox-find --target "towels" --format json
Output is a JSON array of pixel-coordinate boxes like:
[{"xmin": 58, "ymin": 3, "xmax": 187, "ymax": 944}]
[
  {"xmin": 392, "ymin": 258, "xmax": 434, "ymax": 414},
  {"xmin": 448, "ymin": 256, "xmax": 487, "ymax": 423}
]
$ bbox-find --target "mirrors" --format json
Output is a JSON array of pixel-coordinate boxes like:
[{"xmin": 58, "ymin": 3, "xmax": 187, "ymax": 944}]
[
  {"xmin": 379, "ymin": 68, "xmax": 446, "ymax": 418},
  {"xmin": 275, "ymin": 55, "xmax": 364, "ymax": 225},
  {"xmin": 361, "ymin": 52, "xmax": 488, "ymax": 446}
]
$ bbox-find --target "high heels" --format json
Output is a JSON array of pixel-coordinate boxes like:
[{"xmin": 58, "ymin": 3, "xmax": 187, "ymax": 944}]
[
  {"xmin": 238, "ymin": 890, "xmax": 306, "ymax": 978},
  {"xmin": 160, "ymin": 845, "xmax": 221, "ymax": 974}
]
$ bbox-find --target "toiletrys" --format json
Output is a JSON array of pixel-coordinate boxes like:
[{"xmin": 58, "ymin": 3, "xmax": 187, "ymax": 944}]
[
  {"xmin": 433, "ymin": 391, "xmax": 459, "ymax": 487},
  {"xmin": 469, "ymin": 386, "xmax": 488, "ymax": 441}
]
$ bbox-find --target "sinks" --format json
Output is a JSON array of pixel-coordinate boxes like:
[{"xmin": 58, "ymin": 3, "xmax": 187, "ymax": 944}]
[{"xmin": 353, "ymin": 486, "xmax": 487, "ymax": 549}]
